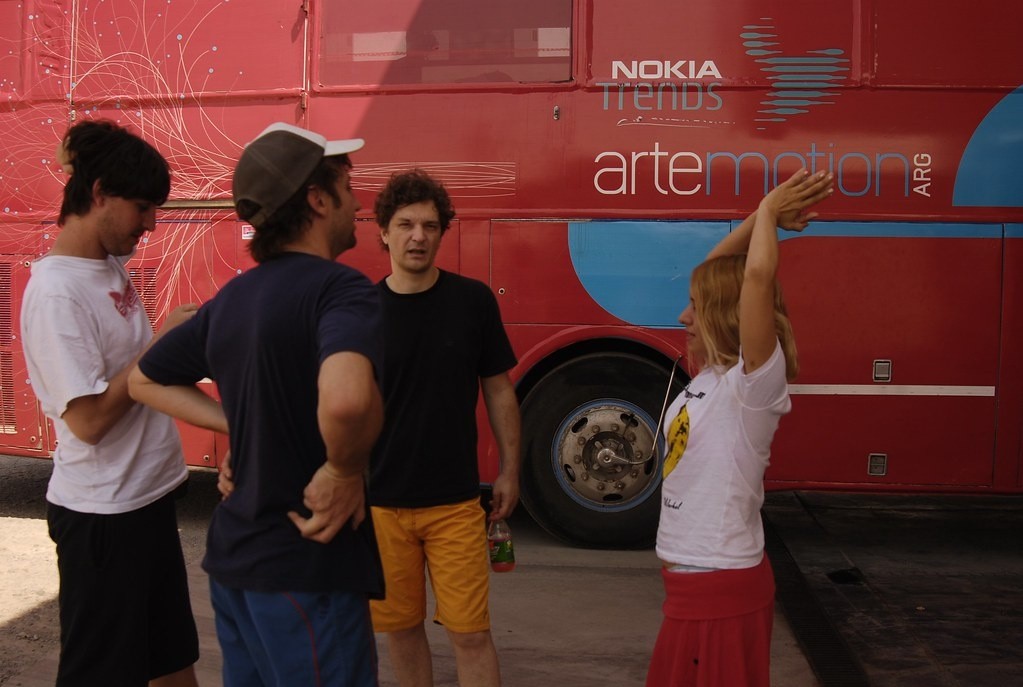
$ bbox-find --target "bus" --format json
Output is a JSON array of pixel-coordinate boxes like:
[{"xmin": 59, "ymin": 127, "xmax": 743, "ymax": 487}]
[{"xmin": 0, "ymin": 0, "xmax": 1023, "ymax": 553}]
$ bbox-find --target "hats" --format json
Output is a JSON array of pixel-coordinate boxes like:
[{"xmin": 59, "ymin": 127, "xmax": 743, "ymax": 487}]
[{"xmin": 231, "ymin": 121, "xmax": 365, "ymax": 226}]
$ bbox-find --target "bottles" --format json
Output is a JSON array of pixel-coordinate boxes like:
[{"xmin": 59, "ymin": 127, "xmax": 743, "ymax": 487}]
[{"xmin": 487, "ymin": 511, "xmax": 517, "ymax": 572}]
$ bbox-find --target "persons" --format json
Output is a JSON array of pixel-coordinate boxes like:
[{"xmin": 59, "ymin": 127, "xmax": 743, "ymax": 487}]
[
  {"xmin": 645, "ymin": 166, "xmax": 836, "ymax": 687},
  {"xmin": 20, "ymin": 119, "xmax": 200, "ymax": 687},
  {"xmin": 368, "ymin": 169, "xmax": 520, "ymax": 687},
  {"xmin": 127, "ymin": 123, "xmax": 389, "ymax": 687}
]
